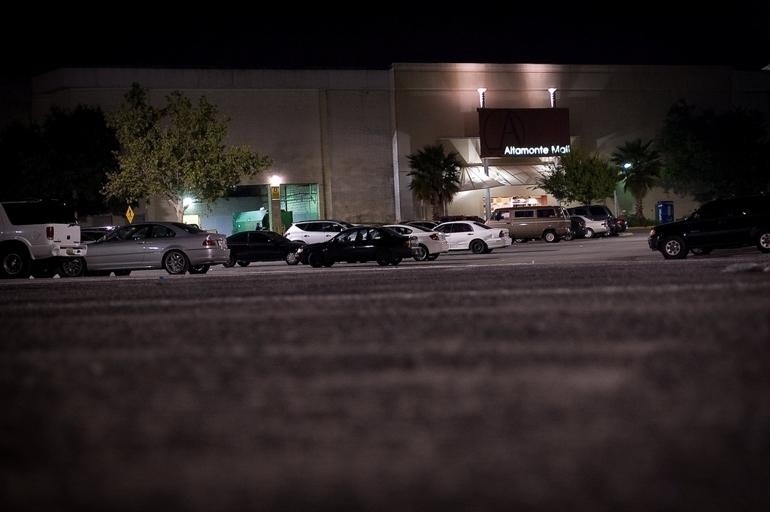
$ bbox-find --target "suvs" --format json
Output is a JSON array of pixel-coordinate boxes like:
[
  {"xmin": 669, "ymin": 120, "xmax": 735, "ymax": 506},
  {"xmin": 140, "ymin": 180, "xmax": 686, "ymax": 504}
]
[
  {"xmin": 284, "ymin": 219, "xmax": 362, "ymax": 245},
  {"xmin": 0, "ymin": 200, "xmax": 130, "ymax": 278}
]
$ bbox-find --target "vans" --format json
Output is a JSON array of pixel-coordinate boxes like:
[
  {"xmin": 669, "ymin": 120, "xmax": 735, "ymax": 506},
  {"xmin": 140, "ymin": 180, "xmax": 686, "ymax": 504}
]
[
  {"xmin": 486, "ymin": 206, "xmax": 572, "ymax": 242},
  {"xmin": 440, "ymin": 215, "xmax": 485, "ymax": 222}
]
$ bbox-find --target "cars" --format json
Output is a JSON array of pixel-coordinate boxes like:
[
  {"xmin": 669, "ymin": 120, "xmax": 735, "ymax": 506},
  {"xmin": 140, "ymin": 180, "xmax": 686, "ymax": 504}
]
[
  {"xmin": 223, "ymin": 231, "xmax": 306, "ymax": 268},
  {"xmin": 514, "ymin": 206, "xmax": 628, "ymax": 243},
  {"xmin": 300, "ymin": 226, "xmax": 412, "ymax": 267},
  {"xmin": 363, "ymin": 223, "xmax": 449, "ymax": 261},
  {"xmin": 648, "ymin": 192, "xmax": 769, "ymax": 260},
  {"xmin": 58, "ymin": 222, "xmax": 231, "ymax": 274},
  {"xmin": 433, "ymin": 221, "xmax": 512, "ymax": 254}
]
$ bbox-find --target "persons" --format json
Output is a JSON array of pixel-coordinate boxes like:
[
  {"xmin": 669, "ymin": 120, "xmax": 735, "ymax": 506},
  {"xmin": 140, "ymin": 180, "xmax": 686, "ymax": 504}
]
[
  {"xmin": 261, "ymin": 209, "xmax": 269, "ymax": 230},
  {"xmin": 255, "ymin": 222, "xmax": 266, "ymax": 232}
]
